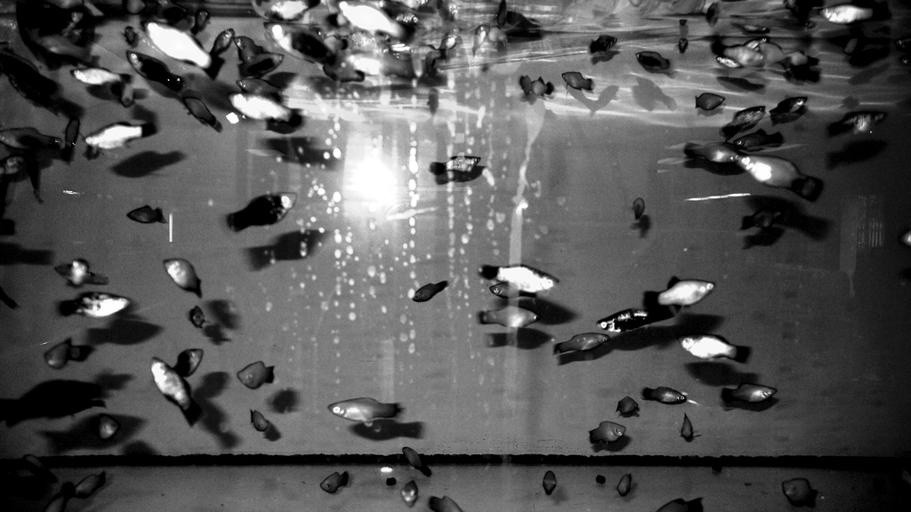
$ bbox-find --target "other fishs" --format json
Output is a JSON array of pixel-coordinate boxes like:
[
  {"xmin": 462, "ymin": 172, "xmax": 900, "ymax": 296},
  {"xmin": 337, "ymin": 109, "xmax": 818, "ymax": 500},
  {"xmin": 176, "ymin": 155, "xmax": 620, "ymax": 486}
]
[{"xmin": 0, "ymin": 0, "xmax": 911, "ymax": 512}]
